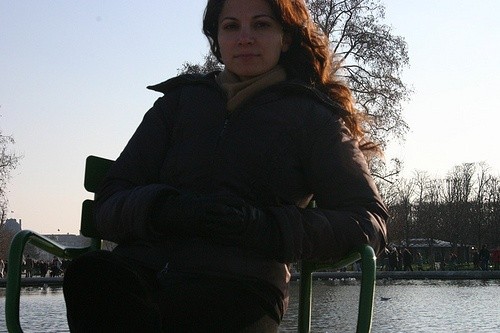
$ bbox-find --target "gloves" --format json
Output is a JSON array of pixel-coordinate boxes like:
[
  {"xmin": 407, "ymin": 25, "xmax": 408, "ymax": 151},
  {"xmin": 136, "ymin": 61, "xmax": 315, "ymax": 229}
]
[{"xmin": 152, "ymin": 188, "xmax": 270, "ymax": 238}]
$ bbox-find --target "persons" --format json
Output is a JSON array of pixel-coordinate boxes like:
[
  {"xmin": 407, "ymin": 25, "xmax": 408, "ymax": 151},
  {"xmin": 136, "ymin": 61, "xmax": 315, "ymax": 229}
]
[
  {"xmin": 366, "ymin": 246, "xmax": 500, "ymax": 272},
  {"xmin": 0, "ymin": 255, "xmax": 64, "ymax": 279},
  {"xmin": 94, "ymin": 0, "xmax": 390, "ymax": 333}
]
